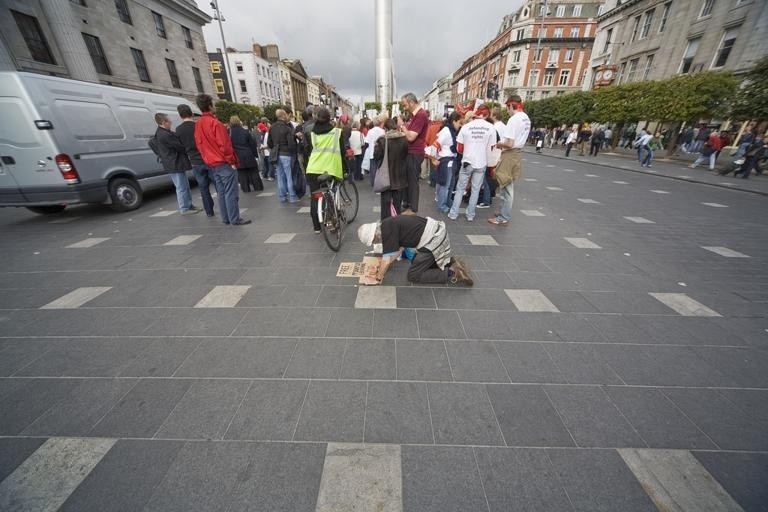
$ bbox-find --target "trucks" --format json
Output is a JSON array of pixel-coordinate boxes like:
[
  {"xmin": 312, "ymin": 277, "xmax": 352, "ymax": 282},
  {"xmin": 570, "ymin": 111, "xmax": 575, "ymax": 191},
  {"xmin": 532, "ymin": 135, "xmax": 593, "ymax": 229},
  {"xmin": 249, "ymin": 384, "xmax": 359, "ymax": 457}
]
[{"xmin": 0, "ymin": 70, "xmax": 211, "ymax": 213}]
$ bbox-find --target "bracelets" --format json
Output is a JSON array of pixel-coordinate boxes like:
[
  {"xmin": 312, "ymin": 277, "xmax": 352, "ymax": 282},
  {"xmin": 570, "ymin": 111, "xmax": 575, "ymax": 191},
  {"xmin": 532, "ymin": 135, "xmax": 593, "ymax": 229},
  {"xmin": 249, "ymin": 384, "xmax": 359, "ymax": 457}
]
[
  {"xmin": 376, "ymin": 266, "xmax": 380, "ymax": 272},
  {"xmin": 401, "ymin": 124, "xmax": 405, "ymax": 129}
]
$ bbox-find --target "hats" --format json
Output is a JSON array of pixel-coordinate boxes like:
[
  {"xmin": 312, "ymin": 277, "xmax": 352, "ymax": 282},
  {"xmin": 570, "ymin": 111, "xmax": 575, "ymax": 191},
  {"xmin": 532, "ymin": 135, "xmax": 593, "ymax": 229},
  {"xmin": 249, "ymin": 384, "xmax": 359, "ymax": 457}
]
[{"xmin": 358, "ymin": 222, "xmax": 378, "ymax": 247}]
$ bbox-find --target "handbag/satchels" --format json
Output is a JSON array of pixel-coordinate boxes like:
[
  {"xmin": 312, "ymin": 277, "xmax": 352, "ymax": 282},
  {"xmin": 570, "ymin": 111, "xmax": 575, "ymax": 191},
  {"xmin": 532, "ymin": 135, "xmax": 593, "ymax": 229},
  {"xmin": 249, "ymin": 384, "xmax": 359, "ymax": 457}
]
[
  {"xmin": 148, "ymin": 130, "xmax": 162, "ymax": 158},
  {"xmin": 292, "ymin": 158, "xmax": 305, "ymax": 198},
  {"xmin": 373, "ymin": 163, "xmax": 391, "ymax": 193}
]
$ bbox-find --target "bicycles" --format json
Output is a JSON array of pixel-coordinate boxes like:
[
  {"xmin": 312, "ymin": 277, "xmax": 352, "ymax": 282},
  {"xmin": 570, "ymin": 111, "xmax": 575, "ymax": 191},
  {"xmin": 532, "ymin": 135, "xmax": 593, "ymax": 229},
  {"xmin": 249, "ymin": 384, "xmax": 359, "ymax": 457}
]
[{"xmin": 308, "ymin": 159, "xmax": 360, "ymax": 251}]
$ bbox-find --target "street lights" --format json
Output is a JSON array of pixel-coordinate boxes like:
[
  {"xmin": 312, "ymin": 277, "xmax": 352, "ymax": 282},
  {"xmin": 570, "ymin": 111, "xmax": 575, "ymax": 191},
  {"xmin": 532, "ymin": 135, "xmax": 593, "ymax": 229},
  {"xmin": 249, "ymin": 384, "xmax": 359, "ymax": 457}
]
[
  {"xmin": 206, "ymin": 0, "xmax": 239, "ymax": 102},
  {"xmin": 526, "ymin": 4, "xmax": 553, "ymax": 112},
  {"xmin": 321, "ymin": 94, "xmax": 331, "ymax": 108}
]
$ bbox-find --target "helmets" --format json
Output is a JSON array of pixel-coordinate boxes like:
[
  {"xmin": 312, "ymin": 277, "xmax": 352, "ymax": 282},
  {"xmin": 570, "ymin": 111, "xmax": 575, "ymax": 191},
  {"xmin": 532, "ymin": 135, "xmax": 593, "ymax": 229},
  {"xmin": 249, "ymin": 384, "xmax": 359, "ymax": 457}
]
[
  {"xmin": 477, "ymin": 104, "xmax": 491, "ymax": 117},
  {"xmin": 505, "ymin": 95, "xmax": 522, "ymax": 105}
]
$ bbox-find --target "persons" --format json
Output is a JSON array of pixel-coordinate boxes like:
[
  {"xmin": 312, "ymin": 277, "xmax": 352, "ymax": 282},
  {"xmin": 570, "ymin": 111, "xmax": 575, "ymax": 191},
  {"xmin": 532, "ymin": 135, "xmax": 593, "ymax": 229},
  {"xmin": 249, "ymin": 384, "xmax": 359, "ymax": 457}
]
[
  {"xmin": 193, "ymin": 95, "xmax": 251, "ymax": 225},
  {"xmin": 688, "ymin": 132, "xmax": 721, "ymax": 170},
  {"xmin": 620, "ymin": 127, "xmax": 673, "ymax": 167},
  {"xmin": 527, "ymin": 123, "xmax": 613, "ymax": 158},
  {"xmin": 373, "ymin": 118, "xmax": 409, "ymax": 221},
  {"xmin": 396, "ymin": 94, "xmax": 429, "ymax": 216},
  {"xmin": 227, "ymin": 105, "xmax": 314, "ymax": 203},
  {"xmin": 420, "ymin": 95, "xmax": 531, "ymax": 224},
  {"xmin": 357, "ymin": 214, "xmax": 474, "ymax": 286},
  {"xmin": 154, "ymin": 113, "xmax": 203, "ymax": 215},
  {"xmin": 174, "ymin": 104, "xmax": 218, "ymax": 217},
  {"xmin": 335, "ymin": 115, "xmax": 388, "ymax": 181},
  {"xmin": 732, "ymin": 126, "xmax": 768, "ymax": 179},
  {"xmin": 303, "ymin": 107, "xmax": 347, "ymax": 234},
  {"xmin": 676, "ymin": 124, "xmax": 731, "ymax": 159}
]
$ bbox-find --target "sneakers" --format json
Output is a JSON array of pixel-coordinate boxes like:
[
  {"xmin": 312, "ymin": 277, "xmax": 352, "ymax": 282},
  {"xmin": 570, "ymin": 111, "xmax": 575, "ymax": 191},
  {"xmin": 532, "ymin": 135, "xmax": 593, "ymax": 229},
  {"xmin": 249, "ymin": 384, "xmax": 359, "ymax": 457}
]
[
  {"xmin": 435, "ymin": 186, "xmax": 513, "ymax": 226},
  {"xmin": 448, "ymin": 255, "xmax": 474, "ymax": 286},
  {"xmin": 181, "ymin": 195, "xmax": 301, "ymax": 225}
]
